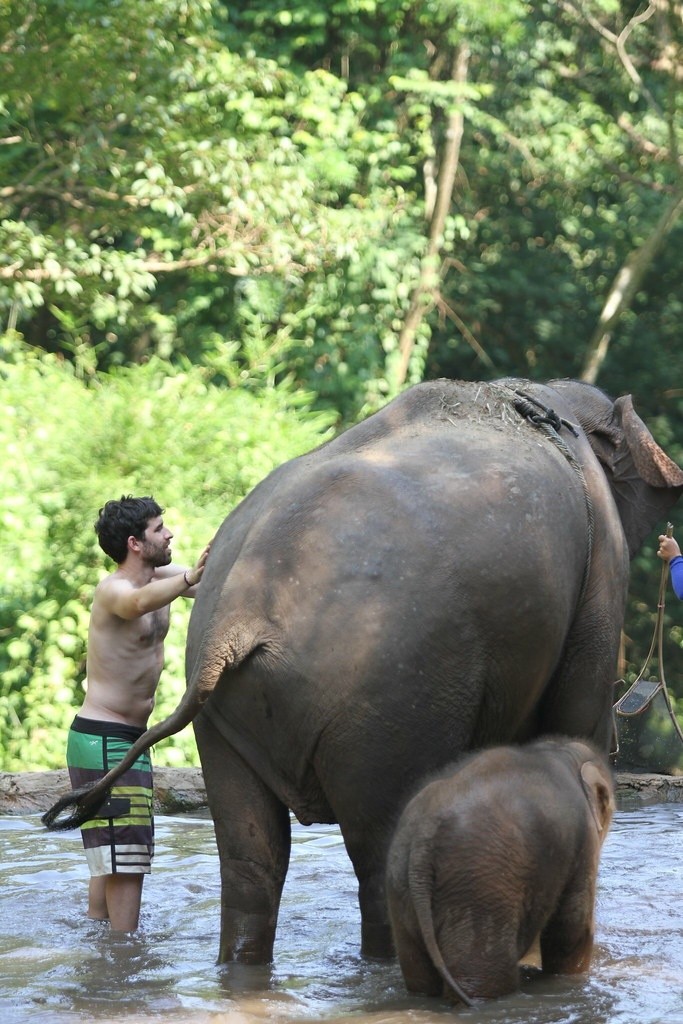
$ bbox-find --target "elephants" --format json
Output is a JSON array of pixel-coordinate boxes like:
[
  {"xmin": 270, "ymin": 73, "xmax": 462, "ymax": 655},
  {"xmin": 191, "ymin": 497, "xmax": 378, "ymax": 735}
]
[
  {"xmin": 385, "ymin": 739, "xmax": 616, "ymax": 1005},
  {"xmin": 45, "ymin": 380, "xmax": 682, "ymax": 964}
]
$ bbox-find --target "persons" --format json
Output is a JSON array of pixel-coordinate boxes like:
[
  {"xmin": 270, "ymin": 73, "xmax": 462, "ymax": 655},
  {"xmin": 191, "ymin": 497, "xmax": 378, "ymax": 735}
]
[
  {"xmin": 657, "ymin": 534, "xmax": 683, "ymax": 601},
  {"xmin": 67, "ymin": 497, "xmax": 210, "ymax": 933}
]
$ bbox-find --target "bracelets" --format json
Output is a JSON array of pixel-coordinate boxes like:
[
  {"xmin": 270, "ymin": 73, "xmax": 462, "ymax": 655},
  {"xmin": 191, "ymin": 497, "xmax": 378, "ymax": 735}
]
[{"xmin": 184, "ymin": 572, "xmax": 192, "ymax": 587}]
